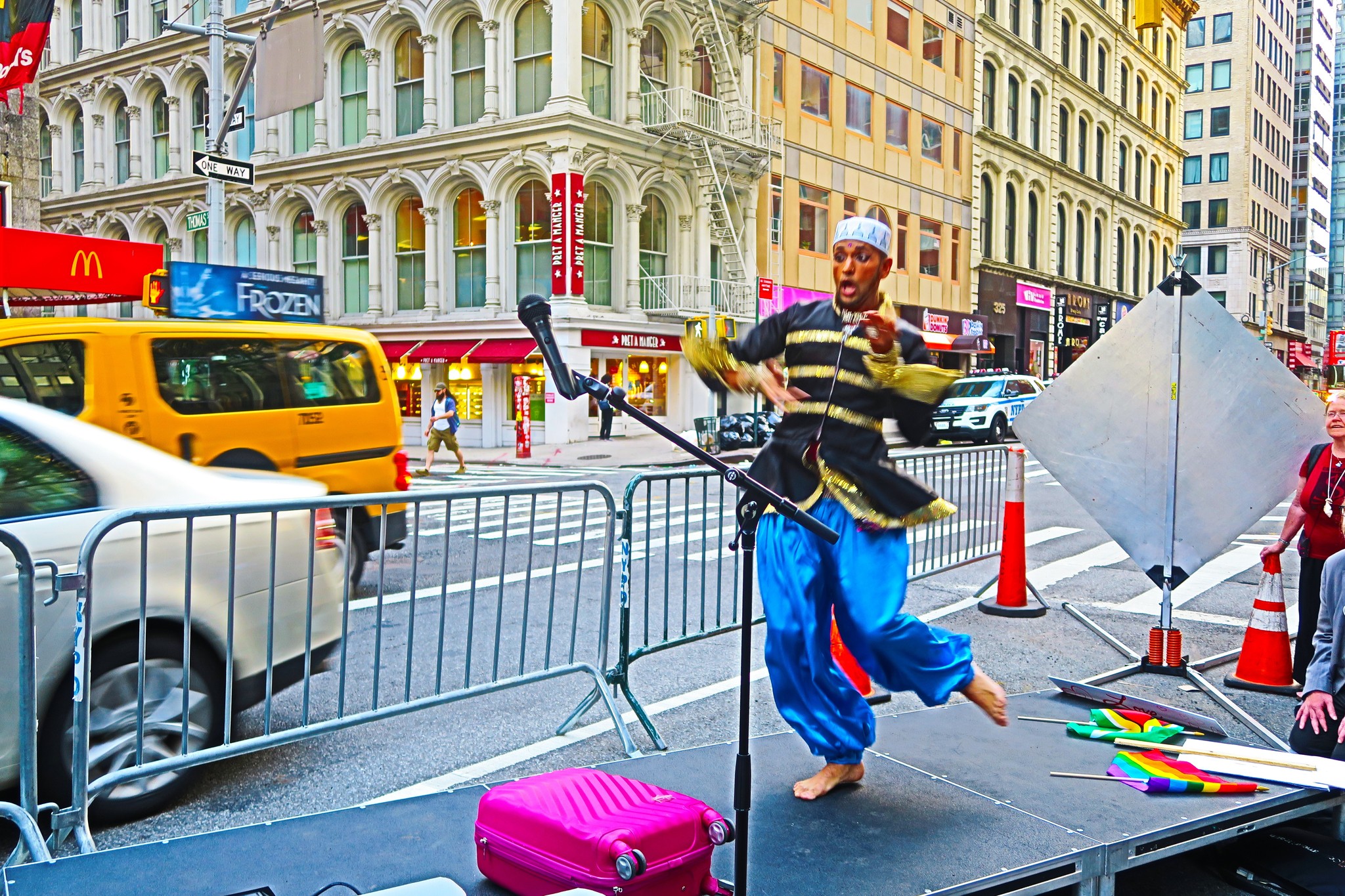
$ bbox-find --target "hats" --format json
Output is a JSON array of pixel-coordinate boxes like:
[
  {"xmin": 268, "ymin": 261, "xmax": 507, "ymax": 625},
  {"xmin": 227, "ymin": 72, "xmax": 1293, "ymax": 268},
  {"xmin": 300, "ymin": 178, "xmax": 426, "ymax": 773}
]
[
  {"xmin": 833, "ymin": 216, "xmax": 891, "ymax": 258},
  {"xmin": 434, "ymin": 382, "xmax": 446, "ymax": 393}
]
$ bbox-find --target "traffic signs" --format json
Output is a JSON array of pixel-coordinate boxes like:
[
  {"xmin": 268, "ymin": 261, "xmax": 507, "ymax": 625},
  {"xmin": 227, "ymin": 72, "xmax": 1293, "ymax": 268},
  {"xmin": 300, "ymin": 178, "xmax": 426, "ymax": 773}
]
[{"xmin": 1264, "ymin": 342, "xmax": 1273, "ymax": 353}]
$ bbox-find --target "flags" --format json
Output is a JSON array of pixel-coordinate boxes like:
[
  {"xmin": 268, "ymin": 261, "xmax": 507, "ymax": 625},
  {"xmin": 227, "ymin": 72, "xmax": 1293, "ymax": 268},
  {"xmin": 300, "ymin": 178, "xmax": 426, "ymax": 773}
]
[{"xmin": 0, "ymin": 0, "xmax": 58, "ymax": 114}]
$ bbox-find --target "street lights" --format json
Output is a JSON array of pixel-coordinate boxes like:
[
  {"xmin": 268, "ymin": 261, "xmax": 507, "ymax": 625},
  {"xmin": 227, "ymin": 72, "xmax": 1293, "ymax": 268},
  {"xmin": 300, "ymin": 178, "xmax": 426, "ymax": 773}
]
[{"xmin": 1263, "ymin": 235, "xmax": 1328, "ymax": 353}]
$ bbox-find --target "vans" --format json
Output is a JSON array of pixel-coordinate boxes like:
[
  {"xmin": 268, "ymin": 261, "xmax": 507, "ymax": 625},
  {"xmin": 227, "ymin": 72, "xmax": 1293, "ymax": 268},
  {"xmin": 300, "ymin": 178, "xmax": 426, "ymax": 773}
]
[{"xmin": 0, "ymin": 316, "xmax": 413, "ymax": 604}]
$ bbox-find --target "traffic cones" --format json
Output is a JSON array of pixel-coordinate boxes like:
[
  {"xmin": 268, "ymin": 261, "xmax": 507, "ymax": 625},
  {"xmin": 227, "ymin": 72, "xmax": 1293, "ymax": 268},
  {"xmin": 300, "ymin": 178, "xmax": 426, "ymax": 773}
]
[{"xmin": 1223, "ymin": 552, "xmax": 1305, "ymax": 696}]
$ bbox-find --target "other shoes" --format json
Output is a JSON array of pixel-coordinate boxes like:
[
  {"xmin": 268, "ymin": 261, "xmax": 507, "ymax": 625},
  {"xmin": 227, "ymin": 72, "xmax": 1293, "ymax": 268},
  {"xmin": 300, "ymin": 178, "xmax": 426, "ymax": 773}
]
[
  {"xmin": 454, "ymin": 466, "xmax": 467, "ymax": 473},
  {"xmin": 415, "ymin": 469, "xmax": 430, "ymax": 476}
]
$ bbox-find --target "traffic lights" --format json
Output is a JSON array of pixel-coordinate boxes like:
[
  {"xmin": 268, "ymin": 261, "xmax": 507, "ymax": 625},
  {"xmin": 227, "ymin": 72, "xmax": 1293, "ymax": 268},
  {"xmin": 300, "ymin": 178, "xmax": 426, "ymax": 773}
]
[{"xmin": 1312, "ymin": 368, "xmax": 1318, "ymax": 374}]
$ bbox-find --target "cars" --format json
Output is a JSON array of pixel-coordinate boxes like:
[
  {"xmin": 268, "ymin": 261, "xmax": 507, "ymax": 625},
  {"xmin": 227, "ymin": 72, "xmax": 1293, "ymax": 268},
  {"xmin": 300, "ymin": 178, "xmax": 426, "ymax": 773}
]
[{"xmin": 0, "ymin": 396, "xmax": 353, "ymax": 825}]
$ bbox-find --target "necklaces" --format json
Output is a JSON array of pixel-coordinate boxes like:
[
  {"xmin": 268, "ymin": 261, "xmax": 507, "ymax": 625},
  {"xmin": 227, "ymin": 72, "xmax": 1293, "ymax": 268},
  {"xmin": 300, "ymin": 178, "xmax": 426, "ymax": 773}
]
[
  {"xmin": 1332, "ymin": 444, "xmax": 1345, "ymax": 467},
  {"xmin": 1323, "ymin": 444, "xmax": 1345, "ymax": 518}
]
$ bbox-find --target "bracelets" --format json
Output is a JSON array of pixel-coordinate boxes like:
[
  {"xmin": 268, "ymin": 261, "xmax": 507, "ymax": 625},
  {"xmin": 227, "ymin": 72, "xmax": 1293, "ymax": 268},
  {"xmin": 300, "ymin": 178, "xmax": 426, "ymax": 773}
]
[{"xmin": 1278, "ymin": 538, "xmax": 1290, "ymax": 546}]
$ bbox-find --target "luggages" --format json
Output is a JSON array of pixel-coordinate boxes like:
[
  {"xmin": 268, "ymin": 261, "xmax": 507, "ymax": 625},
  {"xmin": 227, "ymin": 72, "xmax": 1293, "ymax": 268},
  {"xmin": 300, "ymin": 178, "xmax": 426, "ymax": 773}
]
[{"xmin": 474, "ymin": 768, "xmax": 733, "ymax": 896}]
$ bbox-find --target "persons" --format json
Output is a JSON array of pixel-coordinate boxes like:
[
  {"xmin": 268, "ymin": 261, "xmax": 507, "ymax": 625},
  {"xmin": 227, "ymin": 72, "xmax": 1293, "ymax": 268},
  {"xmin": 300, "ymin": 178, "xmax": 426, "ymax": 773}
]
[
  {"xmin": 1289, "ymin": 498, "xmax": 1345, "ymax": 762},
  {"xmin": 415, "ymin": 382, "xmax": 468, "ymax": 476},
  {"xmin": 684, "ymin": 216, "xmax": 1009, "ymax": 813},
  {"xmin": 340, "ymin": 346, "xmax": 365, "ymax": 398},
  {"xmin": 1259, "ymin": 390, "xmax": 1345, "ymax": 689},
  {"xmin": 598, "ymin": 374, "xmax": 617, "ymax": 441}
]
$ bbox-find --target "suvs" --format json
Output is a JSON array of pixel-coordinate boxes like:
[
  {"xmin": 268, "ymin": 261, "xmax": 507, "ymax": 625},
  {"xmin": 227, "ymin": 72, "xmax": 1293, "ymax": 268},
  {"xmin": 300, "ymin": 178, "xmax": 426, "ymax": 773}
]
[
  {"xmin": 922, "ymin": 368, "xmax": 1046, "ymax": 445},
  {"xmin": 1042, "ymin": 373, "xmax": 1062, "ymax": 388}
]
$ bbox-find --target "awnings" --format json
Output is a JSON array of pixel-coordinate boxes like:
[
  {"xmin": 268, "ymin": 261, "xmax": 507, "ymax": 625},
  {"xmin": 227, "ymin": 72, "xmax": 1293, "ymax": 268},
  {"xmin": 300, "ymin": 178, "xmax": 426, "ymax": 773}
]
[
  {"xmin": 406, "ymin": 338, "xmax": 482, "ymax": 363},
  {"xmin": 947, "ymin": 328, "xmax": 996, "ymax": 358},
  {"xmin": 466, "ymin": 338, "xmax": 540, "ymax": 365},
  {"xmin": 379, "ymin": 340, "xmax": 422, "ymax": 364},
  {"xmin": 919, "ymin": 331, "xmax": 952, "ymax": 354}
]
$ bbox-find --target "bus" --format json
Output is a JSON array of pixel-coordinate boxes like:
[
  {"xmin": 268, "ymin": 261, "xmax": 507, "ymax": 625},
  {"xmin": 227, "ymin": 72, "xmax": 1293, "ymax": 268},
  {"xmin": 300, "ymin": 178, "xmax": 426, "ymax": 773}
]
[{"xmin": 1324, "ymin": 329, "xmax": 1345, "ymax": 406}]
